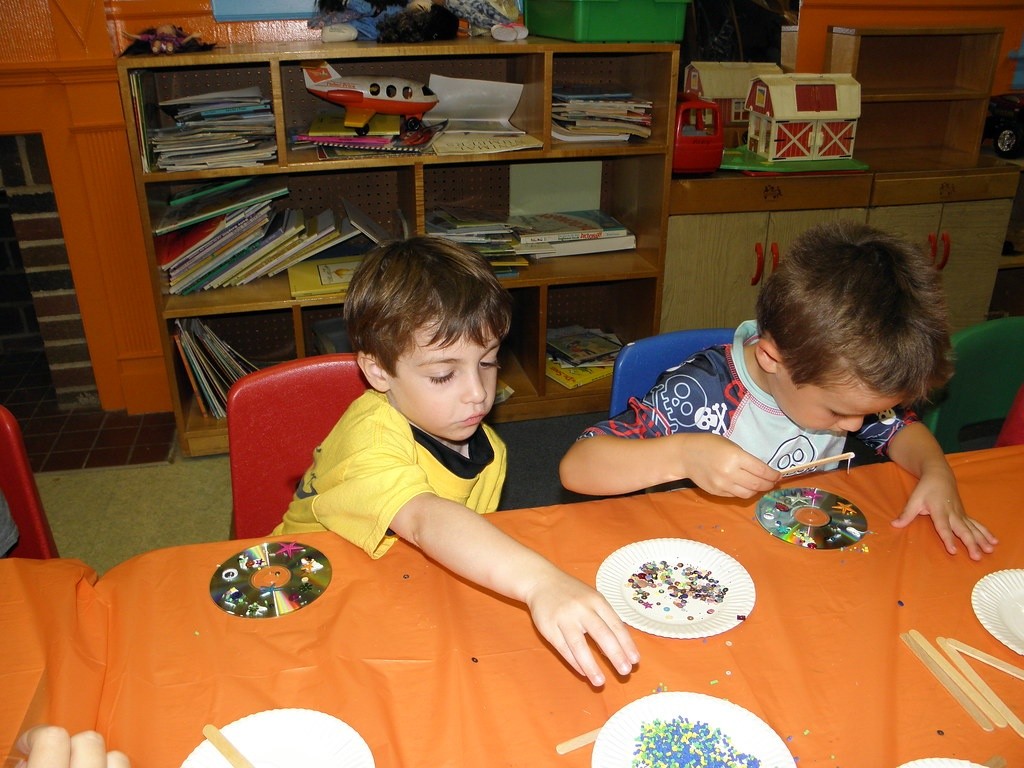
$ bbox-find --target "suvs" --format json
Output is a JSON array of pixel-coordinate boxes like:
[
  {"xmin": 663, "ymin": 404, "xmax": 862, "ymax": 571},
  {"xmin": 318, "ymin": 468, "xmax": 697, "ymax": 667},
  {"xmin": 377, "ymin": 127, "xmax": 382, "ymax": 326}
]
[{"xmin": 979, "ymin": 93, "xmax": 1024, "ymax": 159}]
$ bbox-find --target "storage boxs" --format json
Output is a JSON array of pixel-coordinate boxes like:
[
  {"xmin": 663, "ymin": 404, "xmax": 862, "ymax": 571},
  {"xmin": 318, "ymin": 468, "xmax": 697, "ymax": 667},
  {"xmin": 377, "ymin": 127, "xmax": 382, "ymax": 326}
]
[{"xmin": 523, "ymin": 1, "xmax": 690, "ymax": 46}]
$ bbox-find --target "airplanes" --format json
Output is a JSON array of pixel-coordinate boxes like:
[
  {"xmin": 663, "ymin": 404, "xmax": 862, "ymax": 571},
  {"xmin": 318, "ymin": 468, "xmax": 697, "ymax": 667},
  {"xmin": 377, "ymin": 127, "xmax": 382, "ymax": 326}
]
[{"xmin": 302, "ymin": 62, "xmax": 441, "ymax": 137}]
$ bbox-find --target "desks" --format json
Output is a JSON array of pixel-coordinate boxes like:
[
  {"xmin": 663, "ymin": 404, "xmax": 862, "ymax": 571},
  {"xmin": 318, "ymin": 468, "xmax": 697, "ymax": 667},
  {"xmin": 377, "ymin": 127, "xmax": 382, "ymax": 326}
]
[{"xmin": 0, "ymin": 443, "xmax": 1023, "ymax": 768}]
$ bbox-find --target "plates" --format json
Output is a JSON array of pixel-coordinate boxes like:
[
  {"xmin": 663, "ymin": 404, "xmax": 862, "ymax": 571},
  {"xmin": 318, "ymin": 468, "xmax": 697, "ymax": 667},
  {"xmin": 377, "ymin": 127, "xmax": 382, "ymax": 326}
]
[
  {"xmin": 970, "ymin": 569, "xmax": 1024, "ymax": 655},
  {"xmin": 592, "ymin": 693, "xmax": 796, "ymax": 768},
  {"xmin": 596, "ymin": 537, "xmax": 755, "ymax": 639},
  {"xmin": 179, "ymin": 709, "xmax": 376, "ymax": 768}
]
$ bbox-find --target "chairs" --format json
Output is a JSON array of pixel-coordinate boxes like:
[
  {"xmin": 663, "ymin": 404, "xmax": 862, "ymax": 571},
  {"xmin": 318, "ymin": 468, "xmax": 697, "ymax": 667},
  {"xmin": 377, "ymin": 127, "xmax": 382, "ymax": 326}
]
[
  {"xmin": 608, "ymin": 326, "xmax": 735, "ymax": 418},
  {"xmin": 920, "ymin": 312, "xmax": 1024, "ymax": 453},
  {"xmin": 0, "ymin": 404, "xmax": 64, "ymax": 560},
  {"xmin": 226, "ymin": 351, "xmax": 372, "ymax": 538}
]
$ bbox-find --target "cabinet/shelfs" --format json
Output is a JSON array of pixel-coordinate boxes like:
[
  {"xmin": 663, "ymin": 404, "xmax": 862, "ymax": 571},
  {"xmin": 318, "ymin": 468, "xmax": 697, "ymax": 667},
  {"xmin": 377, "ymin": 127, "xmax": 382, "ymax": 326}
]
[
  {"xmin": 852, "ymin": 144, "xmax": 1024, "ymax": 335},
  {"xmin": 658, "ymin": 173, "xmax": 873, "ymax": 334},
  {"xmin": 115, "ymin": 37, "xmax": 682, "ymax": 459},
  {"xmin": 779, "ymin": 0, "xmax": 1023, "ymax": 171}
]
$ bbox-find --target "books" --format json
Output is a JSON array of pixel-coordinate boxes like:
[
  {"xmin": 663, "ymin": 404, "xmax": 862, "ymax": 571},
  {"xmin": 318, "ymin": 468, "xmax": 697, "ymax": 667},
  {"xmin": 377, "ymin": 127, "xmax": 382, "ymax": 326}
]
[
  {"xmin": 145, "ymin": 84, "xmax": 278, "ymax": 173},
  {"xmin": 167, "ymin": 314, "xmax": 295, "ymax": 421},
  {"xmin": 545, "ymin": 323, "xmax": 627, "ymax": 390},
  {"xmin": 309, "ymin": 316, "xmax": 355, "ymax": 358},
  {"xmin": 550, "ymin": 83, "xmax": 654, "ymax": 143},
  {"xmin": 152, "ymin": 176, "xmax": 412, "ymax": 298},
  {"xmin": 425, "ymin": 205, "xmax": 637, "ymax": 280},
  {"xmin": 290, "ymin": 72, "xmax": 544, "ymax": 159}
]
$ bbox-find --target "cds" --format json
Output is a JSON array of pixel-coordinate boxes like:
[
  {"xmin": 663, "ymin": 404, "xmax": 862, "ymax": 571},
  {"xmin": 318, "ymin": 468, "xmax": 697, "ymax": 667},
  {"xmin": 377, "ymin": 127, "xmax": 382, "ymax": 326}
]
[
  {"xmin": 209, "ymin": 541, "xmax": 332, "ymax": 618},
  {"xmin": 755, "ymin": 488, "xmax": 868, "ymax": 549}
]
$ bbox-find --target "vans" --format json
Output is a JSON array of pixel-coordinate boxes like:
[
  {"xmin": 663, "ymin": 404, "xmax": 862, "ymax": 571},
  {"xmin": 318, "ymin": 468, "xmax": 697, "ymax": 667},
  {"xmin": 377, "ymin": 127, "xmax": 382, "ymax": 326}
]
[{"xmin": 671, "ymin": 92, "xmax": 723, "ymax": 172}]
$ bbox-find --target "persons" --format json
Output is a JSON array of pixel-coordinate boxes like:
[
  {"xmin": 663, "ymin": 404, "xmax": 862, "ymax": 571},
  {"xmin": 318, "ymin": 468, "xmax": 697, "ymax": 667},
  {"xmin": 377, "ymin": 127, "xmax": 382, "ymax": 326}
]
[
  {"xmin": 118, "ymin": 24, "xmax": 220, "ymax": 58},
  {"xmin": 306, "ymin": 0, "xmax": 529, "ymax": 44},
  {"xmin": 558, "ymin": 218, "xmax": 998, "ymax": 562},
  {"xmin": 12, "ymin": 724, "xmax": 133, "ymax": 768},
  {"xmin": 260, "ymin": 230, "xmax": 642, "ymax": 689}
]
